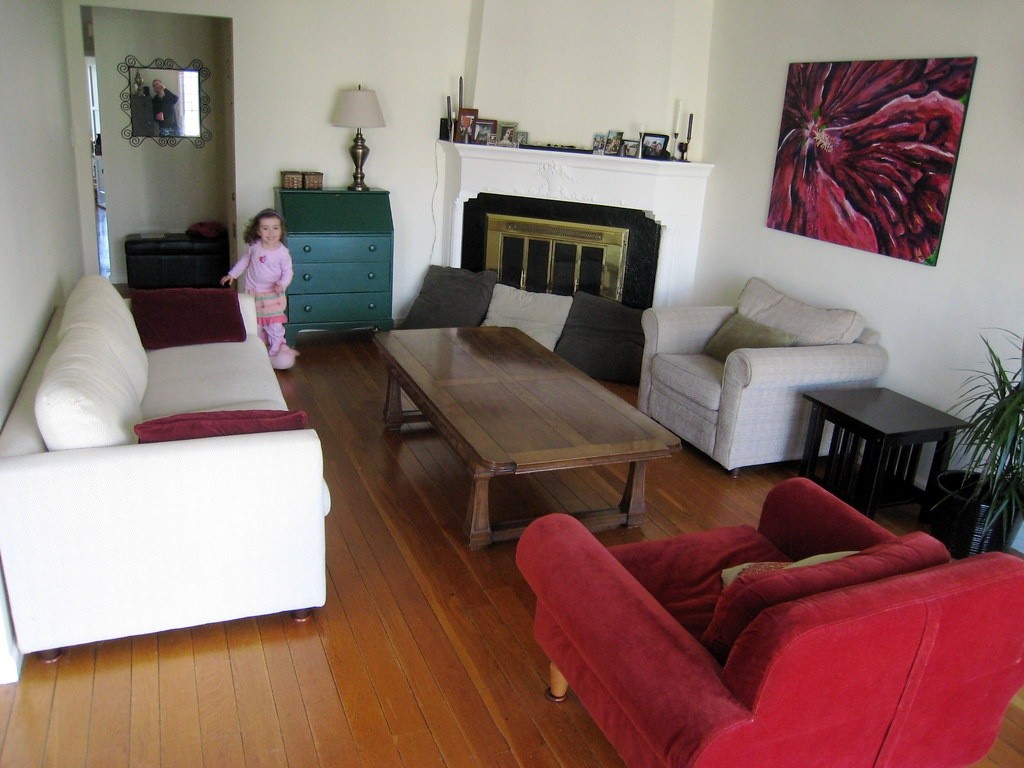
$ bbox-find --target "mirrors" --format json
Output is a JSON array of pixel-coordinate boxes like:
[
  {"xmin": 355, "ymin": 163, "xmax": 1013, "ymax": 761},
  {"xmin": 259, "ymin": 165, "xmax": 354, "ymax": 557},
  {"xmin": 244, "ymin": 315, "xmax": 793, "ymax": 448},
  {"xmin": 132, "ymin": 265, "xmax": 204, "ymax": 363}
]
[{"xmin": 117, "ymin": 55, "xmax": 212, "ymax": 149}]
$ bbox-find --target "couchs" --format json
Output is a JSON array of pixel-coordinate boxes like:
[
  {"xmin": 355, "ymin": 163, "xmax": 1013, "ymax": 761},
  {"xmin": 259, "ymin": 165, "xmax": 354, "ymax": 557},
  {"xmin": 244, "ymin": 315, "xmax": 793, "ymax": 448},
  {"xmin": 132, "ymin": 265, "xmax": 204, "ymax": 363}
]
[
  {"xmin": 0, "ymin": 273, "xmax": 331, "ymax": 664},
  {"xmin": 636, "ymin": 277, "xmax": 886, "ymax": 479}
]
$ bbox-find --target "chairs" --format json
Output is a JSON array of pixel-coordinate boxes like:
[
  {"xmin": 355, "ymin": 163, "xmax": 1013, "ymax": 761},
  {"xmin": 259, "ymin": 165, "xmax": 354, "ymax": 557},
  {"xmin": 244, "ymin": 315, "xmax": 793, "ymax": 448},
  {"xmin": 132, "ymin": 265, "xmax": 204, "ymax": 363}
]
[{"xmin": 515, "ymin": 477, "xmax": 1024, "ymax": 768}]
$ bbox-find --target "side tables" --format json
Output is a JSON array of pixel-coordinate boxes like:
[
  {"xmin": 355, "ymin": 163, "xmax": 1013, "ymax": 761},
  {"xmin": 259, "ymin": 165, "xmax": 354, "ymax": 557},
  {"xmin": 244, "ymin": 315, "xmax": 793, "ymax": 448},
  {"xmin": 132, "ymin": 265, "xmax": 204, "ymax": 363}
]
[{"xmin": 796, "ymin": 388, "xmax": 972, "ymax": 526}]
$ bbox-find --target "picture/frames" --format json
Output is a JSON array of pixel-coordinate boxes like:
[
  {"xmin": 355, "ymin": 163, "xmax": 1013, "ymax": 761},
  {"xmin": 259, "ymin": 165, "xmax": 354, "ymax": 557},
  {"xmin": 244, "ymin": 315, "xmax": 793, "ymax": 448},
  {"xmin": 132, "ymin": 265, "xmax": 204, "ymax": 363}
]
[
  {"xmin": 456, "ymin": 109, "xmax": 478, "ymax": 143},
  {"xmin": 471, "ymin": 119, "xmax": 497, "ymax": 145},
  {"xmin": 496, "ymin": 121, "xmax": 518, "ymax": 146},
  {"xmin": 642, "ymin": 133, "xmax": 669, "ymax": 160}
]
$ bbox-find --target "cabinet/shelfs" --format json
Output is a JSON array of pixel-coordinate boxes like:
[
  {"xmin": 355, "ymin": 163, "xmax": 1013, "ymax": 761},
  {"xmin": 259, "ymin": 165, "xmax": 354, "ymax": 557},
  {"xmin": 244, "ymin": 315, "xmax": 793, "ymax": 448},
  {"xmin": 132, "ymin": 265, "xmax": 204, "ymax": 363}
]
[{"xmin": 273, "ymin": 188, "xmax": 394, "ymax": 349}]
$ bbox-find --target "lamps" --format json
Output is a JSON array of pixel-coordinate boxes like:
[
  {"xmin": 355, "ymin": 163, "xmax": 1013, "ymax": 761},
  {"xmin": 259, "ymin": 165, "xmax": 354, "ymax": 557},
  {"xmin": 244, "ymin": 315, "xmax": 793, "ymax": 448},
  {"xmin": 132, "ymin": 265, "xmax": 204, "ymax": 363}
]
[{"xmin": 333, "ymin": 84, "xmax": 385, "ymax": 191}]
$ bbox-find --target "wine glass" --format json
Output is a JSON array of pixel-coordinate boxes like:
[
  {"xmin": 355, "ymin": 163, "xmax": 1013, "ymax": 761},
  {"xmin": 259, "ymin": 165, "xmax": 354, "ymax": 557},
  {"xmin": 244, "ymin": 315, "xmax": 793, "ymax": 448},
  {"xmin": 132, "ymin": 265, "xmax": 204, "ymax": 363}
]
[{"xmin": 679, "ymin": 142, "xmax": 687, "ymax": 161}]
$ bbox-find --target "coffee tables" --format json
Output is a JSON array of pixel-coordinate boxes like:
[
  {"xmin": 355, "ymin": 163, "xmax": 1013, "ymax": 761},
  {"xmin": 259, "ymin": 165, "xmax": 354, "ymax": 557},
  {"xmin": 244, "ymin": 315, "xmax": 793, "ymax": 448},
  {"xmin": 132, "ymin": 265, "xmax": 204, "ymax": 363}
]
[{"xmin": 371, "ymin": 327, "xmax": 681, "ymax": 553}]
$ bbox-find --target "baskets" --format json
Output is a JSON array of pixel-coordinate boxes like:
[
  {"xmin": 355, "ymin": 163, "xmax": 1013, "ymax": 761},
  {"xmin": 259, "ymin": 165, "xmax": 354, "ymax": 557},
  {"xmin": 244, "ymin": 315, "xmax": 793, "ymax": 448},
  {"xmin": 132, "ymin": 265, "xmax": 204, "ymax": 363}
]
[
  {"xmin": 281, "ymin": 171, "xmax": 303, "ymax": 189},
  {"xmin": 303, "ymin": 171, "xmax": 323, "ymax": 189}
]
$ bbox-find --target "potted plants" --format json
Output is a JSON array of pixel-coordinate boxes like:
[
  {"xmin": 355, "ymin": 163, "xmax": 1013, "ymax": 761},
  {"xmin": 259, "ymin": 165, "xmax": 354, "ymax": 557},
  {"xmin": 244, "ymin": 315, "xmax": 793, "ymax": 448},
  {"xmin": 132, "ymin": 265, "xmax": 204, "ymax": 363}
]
[{"xmin": 929, "ymin": 327, "xmax": 1024, "ymax": 559}]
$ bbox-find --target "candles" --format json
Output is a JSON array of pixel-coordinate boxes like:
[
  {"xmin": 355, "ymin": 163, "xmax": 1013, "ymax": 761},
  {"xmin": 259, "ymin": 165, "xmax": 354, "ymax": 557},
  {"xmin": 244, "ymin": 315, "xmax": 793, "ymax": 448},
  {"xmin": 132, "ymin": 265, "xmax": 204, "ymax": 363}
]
[
  {"xmin": 688, "ymin": 113, "xmax": 693, "ymax": 140},
  {"xmin": 447, "ymin": 96, "xmax": 451, "ymax": 126},
  {"xmin": 675, "ymin": 100, "xmax": 683, "ymax": 133},
  {"xmin": 459, "ymin": 77, "xmax": 463, "ymax": 108}
]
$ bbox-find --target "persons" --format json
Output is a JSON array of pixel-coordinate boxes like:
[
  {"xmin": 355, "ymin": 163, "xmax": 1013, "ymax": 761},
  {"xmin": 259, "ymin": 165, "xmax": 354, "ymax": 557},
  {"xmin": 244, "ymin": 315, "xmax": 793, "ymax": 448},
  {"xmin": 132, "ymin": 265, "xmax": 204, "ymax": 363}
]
[
  {"xmin": 219, "ymin": 206, "xmax": 302, "ymax": 370},
  {"xmin": 642, "ymin": 141, "xmax": 664, "ymax": 157},
  {"xmin": 151, "ymin": 79, "xmax": 181, "ymax": 137}
]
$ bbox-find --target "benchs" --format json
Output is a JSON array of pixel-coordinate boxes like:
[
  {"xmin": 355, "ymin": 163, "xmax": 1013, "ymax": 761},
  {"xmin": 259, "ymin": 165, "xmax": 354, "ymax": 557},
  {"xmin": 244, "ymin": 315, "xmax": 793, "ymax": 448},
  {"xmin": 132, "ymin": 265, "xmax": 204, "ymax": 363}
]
[{"xmin": 125, "ymin": 234, "xmax": 230, "ymax": 288}]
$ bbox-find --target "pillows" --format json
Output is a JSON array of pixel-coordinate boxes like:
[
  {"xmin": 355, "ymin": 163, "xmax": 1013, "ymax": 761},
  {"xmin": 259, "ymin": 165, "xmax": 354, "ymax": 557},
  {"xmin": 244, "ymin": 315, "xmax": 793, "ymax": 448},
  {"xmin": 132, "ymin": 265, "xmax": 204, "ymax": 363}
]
[
  {"xmin": 133, "ymin": 291, "xmax": 246, "ymax": 347},
  {"xmin": 722, "ymin": 551, "xmax": 861, "ymax": 591},
  {"xmin": 399, "ymin": 265, "xmax": 646, "ymax": 385},
  {"xmin": 705, "ymin": 313, "xmax": 798, "ymax": 371},
  {"xmin": 134, "ymin": 410, "xmax": 308, "ymax": 442}
]
